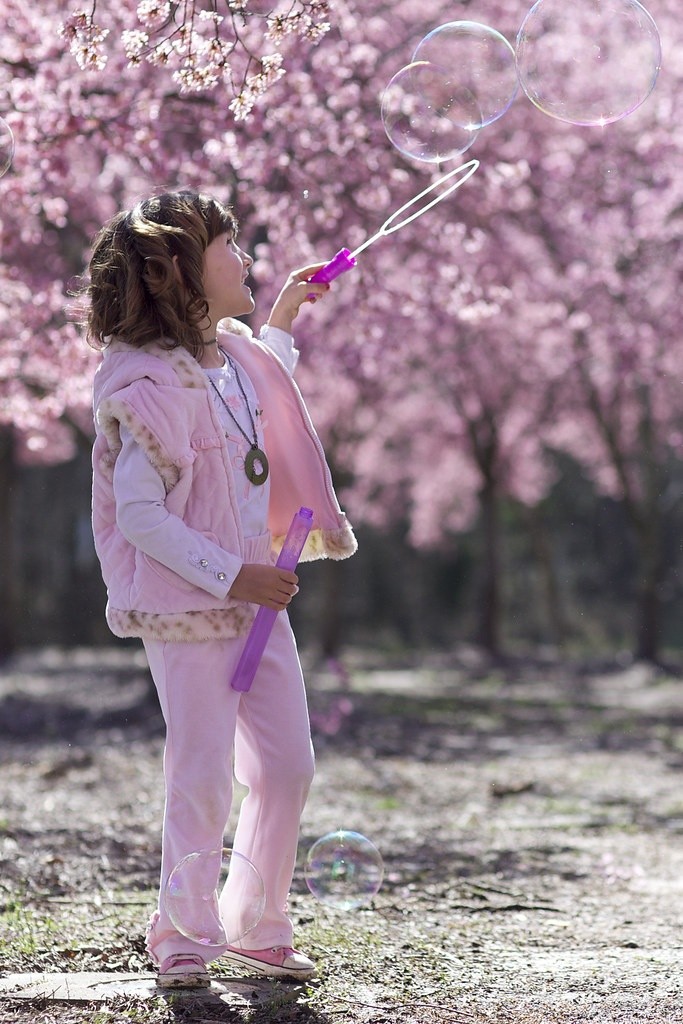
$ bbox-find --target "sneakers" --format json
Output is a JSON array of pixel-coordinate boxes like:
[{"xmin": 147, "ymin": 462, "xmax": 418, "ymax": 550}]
[
  {"xmin": 222, "ymin": 944, "xmax": 318, "ymax": 980},
  {"xmin": 157, "ymin": 954, "xmax": 211, "ymax": 989}
]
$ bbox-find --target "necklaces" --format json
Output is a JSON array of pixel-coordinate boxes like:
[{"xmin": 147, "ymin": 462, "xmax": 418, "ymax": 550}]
[
  {"xmin": 194, "ymin": 337, "xmax": 218, "ymax": 345},
  {"xmin": 207, "ymin": 346, "xmax": 269, "ymax": 486}
]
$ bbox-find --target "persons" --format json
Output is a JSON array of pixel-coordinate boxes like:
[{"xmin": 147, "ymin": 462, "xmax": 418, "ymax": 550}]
[{"xmin": 87, "ymin": 189, "xmax": 359, "ymax": 988}]
